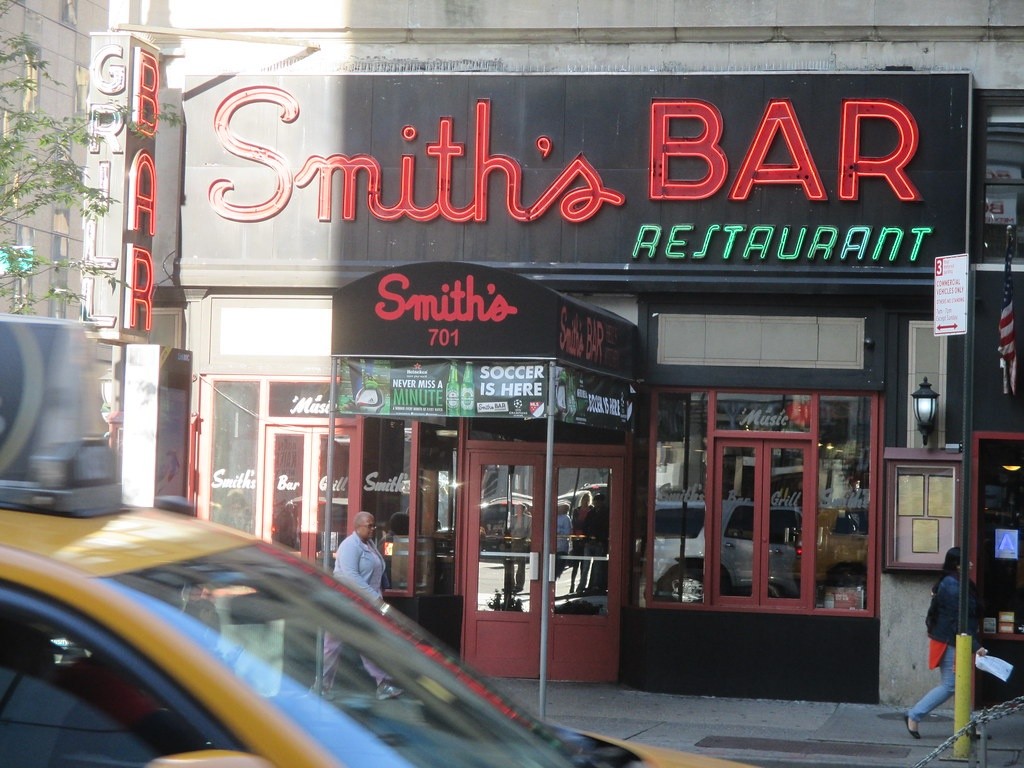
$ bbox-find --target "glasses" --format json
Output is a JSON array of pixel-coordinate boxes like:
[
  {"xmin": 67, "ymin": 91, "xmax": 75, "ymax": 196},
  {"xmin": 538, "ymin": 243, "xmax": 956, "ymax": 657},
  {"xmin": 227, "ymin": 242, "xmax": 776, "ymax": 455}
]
[{"xmin": 359, "ymin": 524, "xmax": 377, "ymax": 529}]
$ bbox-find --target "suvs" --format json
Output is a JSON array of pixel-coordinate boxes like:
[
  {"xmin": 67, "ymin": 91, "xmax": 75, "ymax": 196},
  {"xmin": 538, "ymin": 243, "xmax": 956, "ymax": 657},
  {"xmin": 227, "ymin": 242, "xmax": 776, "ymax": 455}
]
[{"xmin": 578, "ymin": 483, "xmax": 607, "ymax": 499}]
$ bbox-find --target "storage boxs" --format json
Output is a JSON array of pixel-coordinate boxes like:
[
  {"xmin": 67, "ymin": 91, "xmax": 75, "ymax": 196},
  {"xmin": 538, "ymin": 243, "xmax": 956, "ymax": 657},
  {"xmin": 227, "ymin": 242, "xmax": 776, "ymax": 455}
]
[
  {"xmin": 998, "ymin": 611, "xmax": 1015, "ymax": 623},
  {"xmin": 998, "ymin": 622, "xmax": 1015, "ymax": 633}
]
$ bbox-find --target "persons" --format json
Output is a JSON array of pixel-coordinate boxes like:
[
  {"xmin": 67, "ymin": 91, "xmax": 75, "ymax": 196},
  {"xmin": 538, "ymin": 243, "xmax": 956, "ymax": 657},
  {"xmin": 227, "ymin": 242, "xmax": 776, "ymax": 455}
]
[
  {"xmin": 508, "ymin": 502, "xmax": 532, "ymax": 593},
  {"xmin": 557, "ymin": 491, "xmax": 609, "ymax": 593},
  {"xmin": 307, "ymin": 511, "xmax": 403, "ymax": 702},
  {"xmin": 903, "ymin": 547, "xmax": 992, "ymax": 741}
]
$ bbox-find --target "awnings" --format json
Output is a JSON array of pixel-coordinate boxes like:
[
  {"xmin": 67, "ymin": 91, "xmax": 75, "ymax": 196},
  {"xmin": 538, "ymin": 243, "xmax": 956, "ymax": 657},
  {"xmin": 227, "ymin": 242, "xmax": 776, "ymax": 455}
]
[{"xmin": 313, "ymin": 261, "xmax": 644, "ymax": 719}]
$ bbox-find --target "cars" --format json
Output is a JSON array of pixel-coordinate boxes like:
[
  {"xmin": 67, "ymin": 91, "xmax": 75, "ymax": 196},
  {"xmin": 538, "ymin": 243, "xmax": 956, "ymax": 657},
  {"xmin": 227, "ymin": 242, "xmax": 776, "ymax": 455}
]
[
  {"xmin": 479, "ymin": 498, "xmax": 533, "ymax": 552},
  {"xmin": 652, "ymin": 500, "xmax": 803, "ymax": 596},
  {"xmin": 793, "ymin": 507, "xmax": 869, "ymax": 587},
  {"xmin": 0, "ymin": 506, "xmax": 753, "ymax": 768}
]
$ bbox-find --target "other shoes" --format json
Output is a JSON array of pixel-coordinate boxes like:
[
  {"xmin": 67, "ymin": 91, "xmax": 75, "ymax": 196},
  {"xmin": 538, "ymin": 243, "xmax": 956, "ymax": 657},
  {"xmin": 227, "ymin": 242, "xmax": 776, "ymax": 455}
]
[
  {"xmin": 905, "ymin": 716, "xmax": 920, "ymax": 738},
  {"xmin": 976, "ymin": 733, "xmax": 992, "ymax": 739}
]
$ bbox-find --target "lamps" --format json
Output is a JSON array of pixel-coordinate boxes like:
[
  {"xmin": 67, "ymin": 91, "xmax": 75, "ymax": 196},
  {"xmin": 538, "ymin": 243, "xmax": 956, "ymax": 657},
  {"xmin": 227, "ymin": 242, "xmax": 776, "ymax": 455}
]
[{"xmin": 909, "ymin": 376, "xmax": 940, "ymax": 446}]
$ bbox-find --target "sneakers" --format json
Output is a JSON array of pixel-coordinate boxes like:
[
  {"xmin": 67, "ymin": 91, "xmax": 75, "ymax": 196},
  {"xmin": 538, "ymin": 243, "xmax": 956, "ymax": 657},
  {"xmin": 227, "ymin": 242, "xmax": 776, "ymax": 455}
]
[
  {"xmin": 375, "ymin": 682, "xmax": 404, "ymax": 700},
  {"xmin": 309, "ymin": 686, "xmax": 335, "ymax": 701}
]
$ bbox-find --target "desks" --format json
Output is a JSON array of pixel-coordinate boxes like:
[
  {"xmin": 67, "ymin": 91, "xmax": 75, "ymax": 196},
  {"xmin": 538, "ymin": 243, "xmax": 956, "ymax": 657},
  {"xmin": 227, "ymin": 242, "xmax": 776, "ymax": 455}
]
[{"xmin": 983, "ymin": 632, "xmax": 1024, "ymax": 642}]
